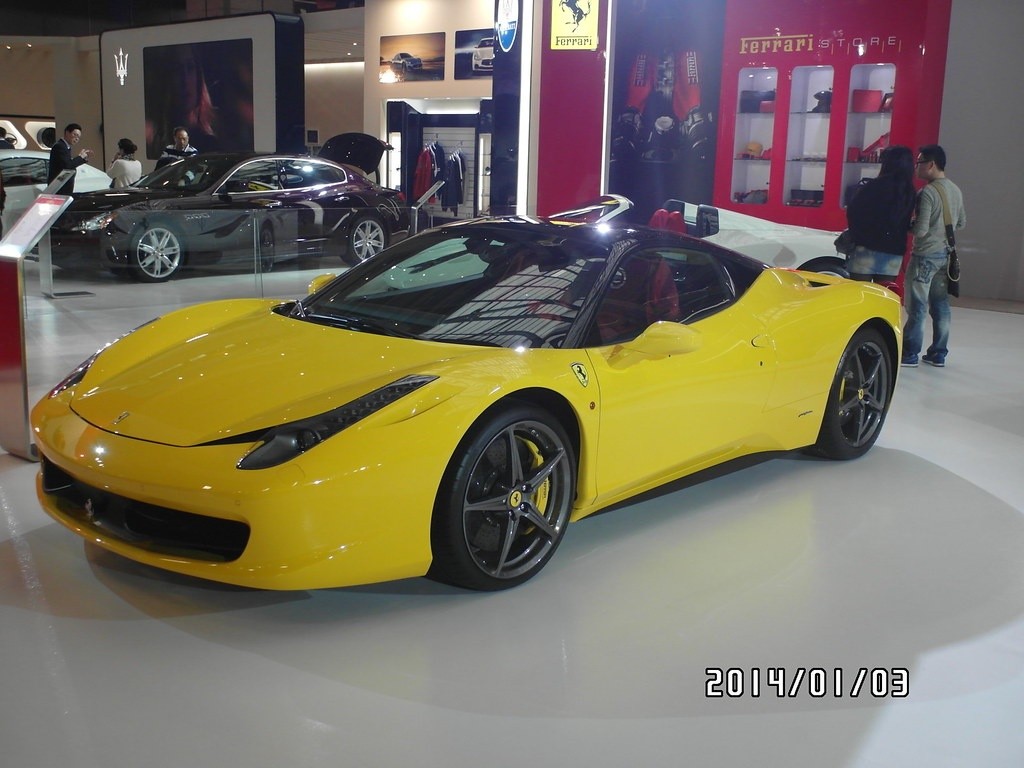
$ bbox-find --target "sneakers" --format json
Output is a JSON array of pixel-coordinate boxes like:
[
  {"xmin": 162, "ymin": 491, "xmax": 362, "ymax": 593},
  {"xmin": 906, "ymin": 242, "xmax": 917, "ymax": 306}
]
[
  {"xmin": 921, "ymin": 353, "xmax": 946, "ymax": 367},
  {"xmin": 900, "ymin": 355, "xmax": 919, "ymax": 366}
]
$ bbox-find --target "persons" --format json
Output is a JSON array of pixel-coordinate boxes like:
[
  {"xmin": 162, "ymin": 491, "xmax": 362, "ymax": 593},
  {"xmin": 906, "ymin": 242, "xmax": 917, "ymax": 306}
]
[
  {"xmin": 0, "ymin": 167, "xmax": 7, "ymax": 241},
  {"xmin": 107, "ymin": 138, "xmax": 142, "ymax": 188},
  {"xmin": 900, "ymin": 145, "xmax": 966, "ymax": 366},
  {"xmin": 848, "ymin": 146, "xmax": 919, "ymax": 291},
  {"xmin": 48, "ymin": 123, "xmax": 95, "ymax": 195},
  {"xmin": 0, "ymin": 127, "xmax": 15, "ymax": 149},
  {"xmin": 154, "ymin": 127, "xmax": 198, "ymax": 186}
]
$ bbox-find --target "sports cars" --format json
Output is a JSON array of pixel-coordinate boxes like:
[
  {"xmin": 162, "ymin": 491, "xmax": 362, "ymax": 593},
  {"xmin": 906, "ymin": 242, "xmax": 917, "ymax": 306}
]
[
  {"xmin": 343, "ymin": 190, "xmax": 847, "ymax": 303},
  {"xmin": 32, "ymin": 222, "xmax": 910, "ymax": 591}
]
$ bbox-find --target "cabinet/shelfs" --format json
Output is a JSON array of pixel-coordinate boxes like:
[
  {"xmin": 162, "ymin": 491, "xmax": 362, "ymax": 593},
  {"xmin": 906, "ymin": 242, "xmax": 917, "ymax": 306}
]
[{"xmin": 732, "ymin": 110, "xmax": 891, "ymax": 205}]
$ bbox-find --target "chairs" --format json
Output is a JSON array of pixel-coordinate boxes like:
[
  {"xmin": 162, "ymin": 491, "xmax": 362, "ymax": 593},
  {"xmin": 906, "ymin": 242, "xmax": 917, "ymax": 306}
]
[
  {"xmin": 597, "ymin": 251, "xmax": 680, "ymax": 344},
  {"xmin": 484, "ymin": 250, "xmax": 567, "ymax": 320},
  {"xmin": 648, "ymin": 210, "xmax": 687, "ymax": 235}
]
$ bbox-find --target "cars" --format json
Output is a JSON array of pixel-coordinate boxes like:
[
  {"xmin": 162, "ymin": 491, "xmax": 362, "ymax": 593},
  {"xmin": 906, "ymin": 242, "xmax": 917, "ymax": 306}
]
[
  {"xmin": 392, "ymin": 52, "xmax": 424, "ymax": 73},
  {"xmin": 53, "ymin": 131, "xmax": 404, "ymax": 275},
  {"xmin": 0, "ymin": 151, "xmax": 113, "ymax": 213},
  {"xmin": 471, "ymin": 38, "xmax": 498, "ymax": 72}
]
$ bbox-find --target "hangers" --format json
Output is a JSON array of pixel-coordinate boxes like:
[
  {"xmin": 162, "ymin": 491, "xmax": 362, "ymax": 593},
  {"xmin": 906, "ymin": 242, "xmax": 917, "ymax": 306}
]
[{"xmin": 422, "ymin": 132, "xmax": 464, "ymax": 163}]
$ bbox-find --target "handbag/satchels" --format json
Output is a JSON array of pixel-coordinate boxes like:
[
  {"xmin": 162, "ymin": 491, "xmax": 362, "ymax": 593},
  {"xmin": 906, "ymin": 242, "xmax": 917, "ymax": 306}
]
[{"xmin": 947, "ymin": 251, "xmax": 959, "ymax": 298}]
